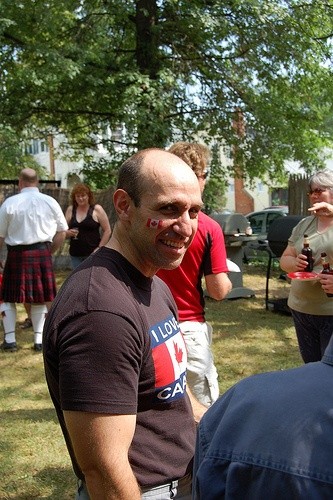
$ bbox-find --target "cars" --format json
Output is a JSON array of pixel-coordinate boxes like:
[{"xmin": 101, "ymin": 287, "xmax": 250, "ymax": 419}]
[{"xmin": 244, "ymin": 206, "xmax": 288, "ymax": 243}]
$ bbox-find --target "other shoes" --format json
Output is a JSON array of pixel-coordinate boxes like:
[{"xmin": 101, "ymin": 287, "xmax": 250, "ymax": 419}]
[
  {"xmin": 0, "ymin": 339, "xmax": 16, "ymax": 351},
  {"xmin": 32, "ymin": 343, "xmax": 42, "ymax": 351},
  {"xmin": 18, "ymin": 317, "xmax": 32, "ymax": 328}
]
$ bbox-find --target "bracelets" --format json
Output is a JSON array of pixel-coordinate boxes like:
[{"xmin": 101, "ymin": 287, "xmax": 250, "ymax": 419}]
[{"xmin": 99, "ymin": 246, "xmax": 100, "ymax": 248}]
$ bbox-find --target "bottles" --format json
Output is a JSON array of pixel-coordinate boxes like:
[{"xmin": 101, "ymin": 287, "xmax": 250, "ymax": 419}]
[
  {"xmin": 320, "ymin": 252, "xmax": 333, "ymax": 297},
  {"xmin": 302, "ymin": 234, "xmax": 314, "ymax": 272},
  {"xmin": 246, "ymin": 222, "xmax": 252, "ymax": 237}
]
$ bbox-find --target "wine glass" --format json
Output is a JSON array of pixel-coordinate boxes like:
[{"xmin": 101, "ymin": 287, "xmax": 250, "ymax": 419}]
[{"xmin": 72, "ymin": 227, "xmax": 79, "ymax": 239}]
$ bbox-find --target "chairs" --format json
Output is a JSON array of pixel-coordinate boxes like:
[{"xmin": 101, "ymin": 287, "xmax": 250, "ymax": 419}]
[{"xmin": 247, "ymin": 215, "xmax": 306, "ymax": 311}]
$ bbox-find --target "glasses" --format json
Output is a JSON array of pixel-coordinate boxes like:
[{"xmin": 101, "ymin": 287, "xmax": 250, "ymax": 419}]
[
  {"xmin": 306, "ymin": 188, "xmax": 327, "ymax": 196},
  {"xmin": 195, "ymin": 171, "xmax": 209, "ymax": 180}
]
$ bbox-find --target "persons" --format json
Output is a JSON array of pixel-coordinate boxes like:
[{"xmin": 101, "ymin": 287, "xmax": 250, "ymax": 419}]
[
  {"xmin": 42, "ymin": 147, "xmax": 210, "ymax": 500},
  {"xmin": 0, "ymin": 140, "xmax": 333, "ymax": 500}
]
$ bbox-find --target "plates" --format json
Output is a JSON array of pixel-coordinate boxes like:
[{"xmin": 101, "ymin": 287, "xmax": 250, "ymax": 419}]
[{"xmin": 288, "ymin": 272, "xmax": 319, "ymax": 281}]
